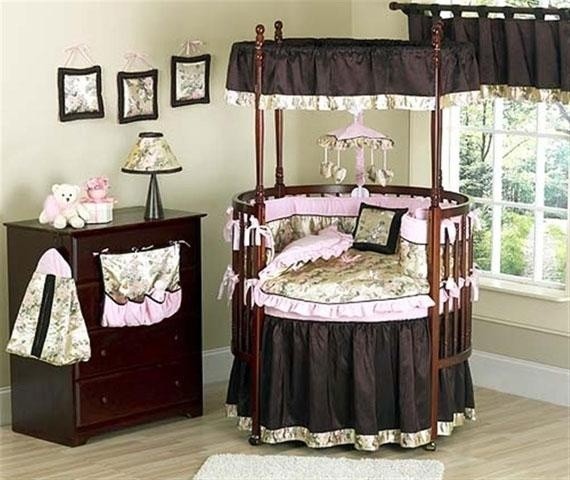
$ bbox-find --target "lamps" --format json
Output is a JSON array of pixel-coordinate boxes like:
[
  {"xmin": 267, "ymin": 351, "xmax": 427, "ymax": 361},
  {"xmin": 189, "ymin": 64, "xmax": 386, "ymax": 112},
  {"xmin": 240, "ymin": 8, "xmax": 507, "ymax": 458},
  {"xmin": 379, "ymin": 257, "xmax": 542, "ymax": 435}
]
[{"xmin": 120, "ymin": 131, "xmax": 183, "ymax": 220}]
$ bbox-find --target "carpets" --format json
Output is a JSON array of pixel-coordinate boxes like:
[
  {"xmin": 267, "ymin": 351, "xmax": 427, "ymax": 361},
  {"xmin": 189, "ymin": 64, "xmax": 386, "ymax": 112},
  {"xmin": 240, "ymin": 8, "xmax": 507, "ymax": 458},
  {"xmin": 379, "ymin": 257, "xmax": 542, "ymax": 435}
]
[{"xmin": 191, "ymin": 453, "xmax": 444, "ymax": 480}]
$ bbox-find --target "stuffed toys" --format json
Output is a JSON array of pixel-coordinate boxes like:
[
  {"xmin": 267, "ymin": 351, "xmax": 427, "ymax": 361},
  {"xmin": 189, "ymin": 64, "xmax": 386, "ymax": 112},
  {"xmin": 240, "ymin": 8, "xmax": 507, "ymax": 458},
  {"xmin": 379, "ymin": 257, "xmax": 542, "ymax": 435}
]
[{"xmin": 39, "ymin": 184, "xmax": 90, "ymax": 229}]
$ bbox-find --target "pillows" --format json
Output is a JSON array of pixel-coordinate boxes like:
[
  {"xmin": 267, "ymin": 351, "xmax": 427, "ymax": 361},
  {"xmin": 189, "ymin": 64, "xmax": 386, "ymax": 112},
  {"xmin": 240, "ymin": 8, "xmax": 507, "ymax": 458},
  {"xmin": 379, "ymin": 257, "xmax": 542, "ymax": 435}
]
[{"xmin": 350, "ymin": 203, "xmax": 409, "ymax": 255}]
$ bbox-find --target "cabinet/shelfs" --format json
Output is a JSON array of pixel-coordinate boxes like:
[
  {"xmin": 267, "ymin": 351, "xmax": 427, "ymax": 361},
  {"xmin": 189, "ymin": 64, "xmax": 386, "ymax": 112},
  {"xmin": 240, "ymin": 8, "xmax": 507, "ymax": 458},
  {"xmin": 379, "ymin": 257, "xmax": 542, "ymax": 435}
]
[{"xmin": 2, "ymin": 205, "xmax": 207, "ymax": 447}]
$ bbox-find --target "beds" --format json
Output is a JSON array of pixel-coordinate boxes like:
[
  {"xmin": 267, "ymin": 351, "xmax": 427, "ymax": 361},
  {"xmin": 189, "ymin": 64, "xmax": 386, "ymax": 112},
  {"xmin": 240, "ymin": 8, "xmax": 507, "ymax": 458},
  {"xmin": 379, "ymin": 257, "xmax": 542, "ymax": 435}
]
[{"xmin": 217, "ymin": 20, "xmax": 480, "ymax": 452}]
